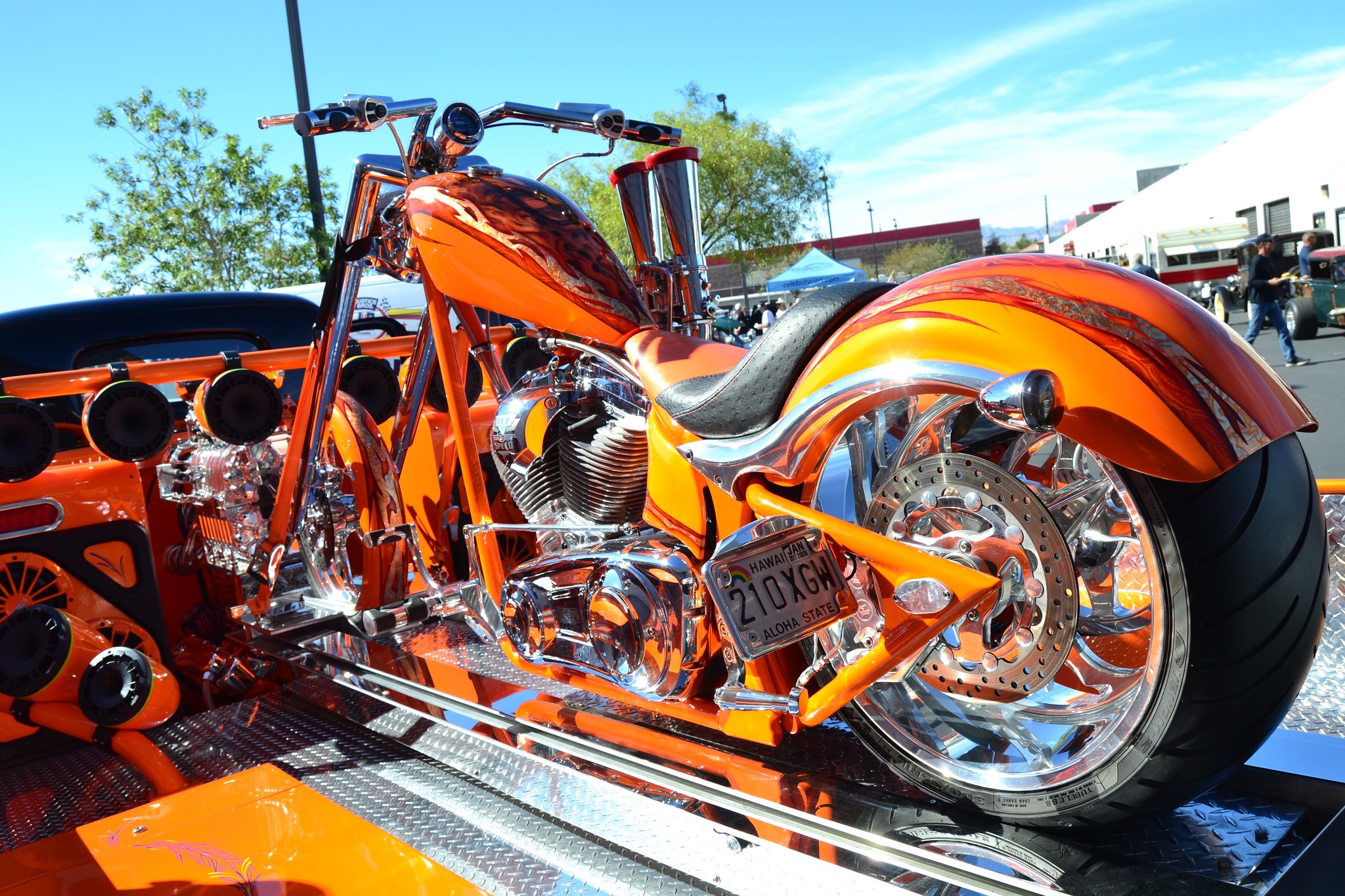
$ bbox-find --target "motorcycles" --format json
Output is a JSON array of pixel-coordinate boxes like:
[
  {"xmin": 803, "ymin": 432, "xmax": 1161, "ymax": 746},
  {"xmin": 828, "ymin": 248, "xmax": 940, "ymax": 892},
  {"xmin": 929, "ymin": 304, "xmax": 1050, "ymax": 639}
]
[{"xmin": 231, "ymin": 89, "xmax": 1331, "ymax": 829}]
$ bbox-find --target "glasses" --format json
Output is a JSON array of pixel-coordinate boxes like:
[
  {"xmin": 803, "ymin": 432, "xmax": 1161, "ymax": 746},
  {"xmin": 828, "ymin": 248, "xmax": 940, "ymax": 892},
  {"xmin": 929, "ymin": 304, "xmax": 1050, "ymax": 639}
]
[{"xmin": 1265, "ymin": 239, "xmax": 1275, "ymax": 242}]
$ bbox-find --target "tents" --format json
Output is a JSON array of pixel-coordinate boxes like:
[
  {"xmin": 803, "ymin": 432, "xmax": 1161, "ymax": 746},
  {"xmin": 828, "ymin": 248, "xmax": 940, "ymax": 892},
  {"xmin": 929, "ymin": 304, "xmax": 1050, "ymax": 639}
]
[{"xmin": 764, "ymin": 247, "xmax": 867, "ymax": 304}]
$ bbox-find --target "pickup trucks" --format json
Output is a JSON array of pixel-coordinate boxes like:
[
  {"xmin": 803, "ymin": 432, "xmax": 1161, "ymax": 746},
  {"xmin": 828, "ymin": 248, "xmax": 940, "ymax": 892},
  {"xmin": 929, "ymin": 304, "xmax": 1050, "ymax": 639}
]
[{"xmin": 0, "ymin": 300, "xmax": 1343, "ymax": 895}]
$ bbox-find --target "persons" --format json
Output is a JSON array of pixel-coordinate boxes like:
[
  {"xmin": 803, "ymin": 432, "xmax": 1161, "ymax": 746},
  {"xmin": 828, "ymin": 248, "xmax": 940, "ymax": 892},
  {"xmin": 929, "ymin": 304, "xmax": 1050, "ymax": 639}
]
[
  {"xmin": 1242, "ymin": 233, "xmax": 1311, "ymax": 367},
  {"xmin": 726, "ymin": 298, "xmax": 794, "ymax": 336},
  {"xmin": 1129, "ymin": 253, "xmax": 1161, "ymax": 283},
  {"xmin": 1298, "ymin": 231, "xmax": 1317, "ymax": 279}
]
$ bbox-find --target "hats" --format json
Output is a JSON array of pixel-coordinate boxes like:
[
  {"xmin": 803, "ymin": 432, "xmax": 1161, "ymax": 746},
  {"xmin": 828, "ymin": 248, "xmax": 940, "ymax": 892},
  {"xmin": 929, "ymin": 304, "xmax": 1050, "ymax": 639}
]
[{"xmin": 1256, "ymin": 234, "xmax": 1280, "ymax": 244}]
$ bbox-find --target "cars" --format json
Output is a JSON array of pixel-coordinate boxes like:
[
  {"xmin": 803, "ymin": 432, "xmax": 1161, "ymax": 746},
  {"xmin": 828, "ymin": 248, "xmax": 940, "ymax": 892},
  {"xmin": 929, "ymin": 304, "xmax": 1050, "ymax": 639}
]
[
  {"xmin": 1286, "ymin": 246, "xmax": 1345, "ymax": 337},
  {"xmin": 1213, "ymin": 229, "xmax": 1334, "ymax": 329}
]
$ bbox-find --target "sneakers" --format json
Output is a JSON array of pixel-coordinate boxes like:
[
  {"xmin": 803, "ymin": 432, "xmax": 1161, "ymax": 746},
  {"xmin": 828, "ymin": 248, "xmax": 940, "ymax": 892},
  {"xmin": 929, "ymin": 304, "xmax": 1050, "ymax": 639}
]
[{"xmin": 1285, "ymin": 356, "xmax": 1311, "ymax": 368}]
